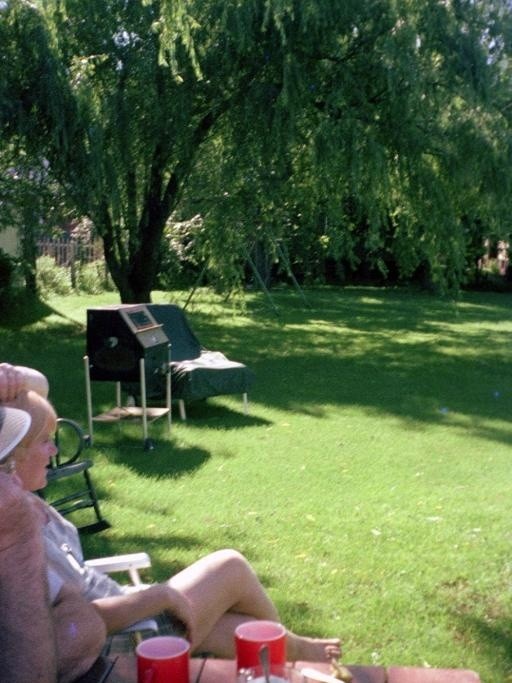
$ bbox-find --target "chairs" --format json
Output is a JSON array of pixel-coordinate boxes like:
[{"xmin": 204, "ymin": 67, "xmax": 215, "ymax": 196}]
[
  {"xmin": 127, "ymin": 301, "xmax": 254, "ymax": 419},
  {"xmin": 76, "ymin": 548, "xmax": 214, "ymax": 659},
  {"xmin": 36, "ymin": 413, "xmax": 110, "ymax": 538}
]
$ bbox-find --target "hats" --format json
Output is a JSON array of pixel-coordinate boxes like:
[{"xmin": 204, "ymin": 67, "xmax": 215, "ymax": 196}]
[{"xmin": 0, "ymin": 405, "xmax": 32, "ymax": 461}]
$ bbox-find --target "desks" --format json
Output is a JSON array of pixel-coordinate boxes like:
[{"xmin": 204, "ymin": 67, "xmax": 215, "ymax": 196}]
[
  {"xmin": 81, "ymin": 343, "xmax": 176, "ymax": 450},
  {"xmin": 76, "ymin": 646, "xmax": 481, "ymax": 681}
]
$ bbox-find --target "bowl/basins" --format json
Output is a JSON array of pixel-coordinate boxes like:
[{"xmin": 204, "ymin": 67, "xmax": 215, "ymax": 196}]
[{"xmin": 300, "ymin": 667, "xmax": 345, "ymax": 682}]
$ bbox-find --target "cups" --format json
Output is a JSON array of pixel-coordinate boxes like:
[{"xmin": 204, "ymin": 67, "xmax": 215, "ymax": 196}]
[
  {"xmin": 238, "ymin": 665, "xmax": 306, "ymax": 683},
  {"xmin": 235, "ymin": 621, "xmax": 285, "ymax": 678},
  {"xmin": 134, "ymin": 635, "xmax": 190, "ymax": 682}
]
